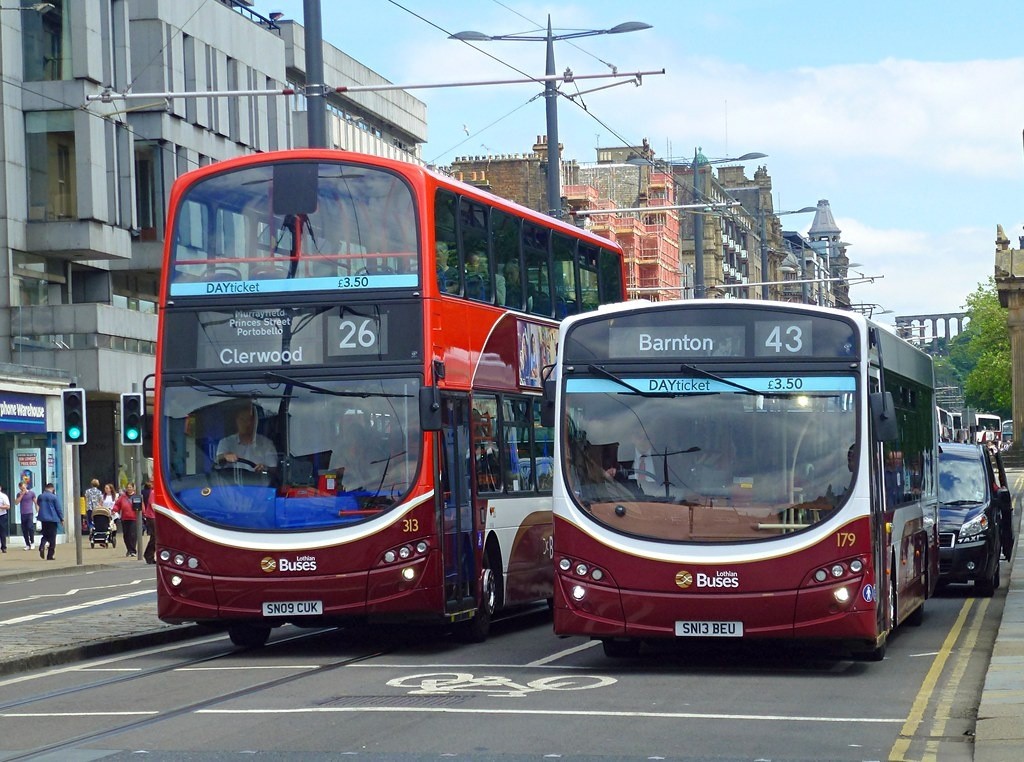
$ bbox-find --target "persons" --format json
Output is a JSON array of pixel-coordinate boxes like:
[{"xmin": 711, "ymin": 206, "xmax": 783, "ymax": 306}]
[
  {"xmin": 215, "ymin": 406, "xmax": 278, "ymax": 473},
  {"xmin": 518, "ymin": 319, "xmax": 558, "ymax": 387},
  {"xmin": 467, "ymin": 250, "xmax": 506, "ymax": 306},
  {"xmin": 99, "ymin": 484, "xmax": 121, "ymax": 549},
  {"xmin": 503, "ymin": 259, "xmax": 533, "ymax": 311},
  {"xmin": 14, "ymin": 482, "xmax": 39, "ymax": 551},
  {"xmin": 36, "ymin": 483, "xmax": 64, "ymax": 560},
  {"xmin": 84, "ymin": 479, "xmax": 103, "ymax": 542},
  {"xmin": 141, "ymin": 482, "xmax": 152, "ymax": 537},
  {"xmin": 0, "ymin": 486, "xmax": 11, "ymax": 554},
  {"xmin": 436, "ymin": 242, "xmax": 460, "ymax": 296},
  {"xmin": 145, "ymin": 490, "xmax": 156, "ymax": 565},
  {"xmin": 112, "ymin": 483, "xmax": 138, "ymax": 556},
  {"xmin": 604, "ymin": 421, "xmax": 684, "ymax": 490}
]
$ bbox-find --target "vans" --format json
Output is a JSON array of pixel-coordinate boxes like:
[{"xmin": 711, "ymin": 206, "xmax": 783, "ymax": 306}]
[{"xmin": 935, "ymin": 441, "xmax": 1014, "ymax": 599}]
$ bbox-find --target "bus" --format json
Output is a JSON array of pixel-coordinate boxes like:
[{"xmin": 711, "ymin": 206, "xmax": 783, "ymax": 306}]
[
  {"xmin": 545, "ymin": 299, "xmax": 942, "ymax": 663},
  {"xmin": 139, "ymin": 145, "xmax": 633, "ymax": 654},
  {"xmin": 936, "ymin": 405, "xmax": 1013, "ymax": 442}
]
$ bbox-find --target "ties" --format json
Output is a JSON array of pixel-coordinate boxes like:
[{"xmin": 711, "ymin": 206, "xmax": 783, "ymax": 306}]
[{"xmin": 638, "ymin": 455, "xmax": 646, "ymax": 480}]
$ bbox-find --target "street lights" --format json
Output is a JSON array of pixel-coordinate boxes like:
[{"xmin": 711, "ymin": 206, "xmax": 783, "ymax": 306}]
[
  {"xmin": 778, "ymin": 262, "xmax": 863, "ymax": 307},
  {"xmin": 630, "ymin": 149, "xmax": 770, "ymax": 299},
  {"xmin": 863, "ymin": 308, "xmax": 892, "ymax": 319},
  {"xmin": 720, "ymin": 207, "xmax": 819, "ymax": 301},
  {"xmin": 449, "ymin": 19, "xmax": 652, "ymax": 220}
]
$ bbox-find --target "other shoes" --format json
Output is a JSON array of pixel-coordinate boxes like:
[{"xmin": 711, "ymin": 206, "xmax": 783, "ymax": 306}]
[
  {"xmin": 47, "ymin": 556, "xmax": 55, "ymax": 560},
  {"xmin": 2, "ymin": 548, "xmax": 7, "ymax": 553},
  {"xmin": 89, "ymin": 534, "xmax": 92, "ymax": 541},
  {"xmin": 100, "ymin": 543, "xmax": 105, "ymax": 547},
  {"xmin": 30, "ymin": 544, "xmax": 35, "ymax": 549},
  {"xmin": 24, "ymin": 545, "xmax": 30, "ymax": 550},
  {"xmin": 40, "ymin": 548, "xmax": 44, "ymax": 559},
  {"xmin": 126, "ymin": 552, "xmax": 136, "ymax": 557}
]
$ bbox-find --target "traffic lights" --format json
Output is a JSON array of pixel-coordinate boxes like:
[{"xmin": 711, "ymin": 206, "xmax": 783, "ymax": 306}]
[
  {"xmin": 60, "ymin": 388, "xmax": 88, "ymax": 445},
  {"xmin": 119, "ymin": 392, "xmax": 144, "ymax": 447}
]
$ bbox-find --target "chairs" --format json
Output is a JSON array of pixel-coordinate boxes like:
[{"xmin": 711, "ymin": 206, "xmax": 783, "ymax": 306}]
[{"xmin": 191, "ymin": 254, "xmax": 597, "ymax": 330}]
